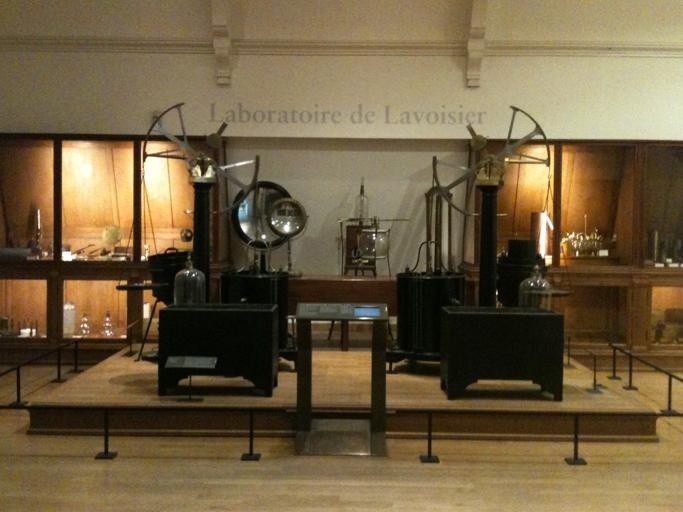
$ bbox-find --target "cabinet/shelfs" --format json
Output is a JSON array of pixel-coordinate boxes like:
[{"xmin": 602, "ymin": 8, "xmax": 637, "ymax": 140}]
[
  {"xmin": 476, "ymin": 138, "xmax": 683, "ymax": 359},
  {"xmin": 0, "ymin": 132, "xmax": 220, "ymax": 364}
]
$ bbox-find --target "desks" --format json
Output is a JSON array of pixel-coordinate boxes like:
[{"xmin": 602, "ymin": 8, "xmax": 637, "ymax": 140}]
[
  {"xmin": 157, "ymin": 303, "xmax": 279, "ymax": 398},
  {"xmin": 283, "ymin": 274, "xmax": 399, "ymax": 351},
  {"xmin": 438, "ymin": 305, "xmax": 566, "ymax": 401}
]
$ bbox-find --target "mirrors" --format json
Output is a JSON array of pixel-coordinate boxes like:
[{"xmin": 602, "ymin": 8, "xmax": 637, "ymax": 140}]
[
  {"xmin": 231, "ymin": 180, "xmax": 294, "ymax": 278},
  {"xmin": 267, "ymin": 198, "xmax": 308, "ymax": 278}
]
